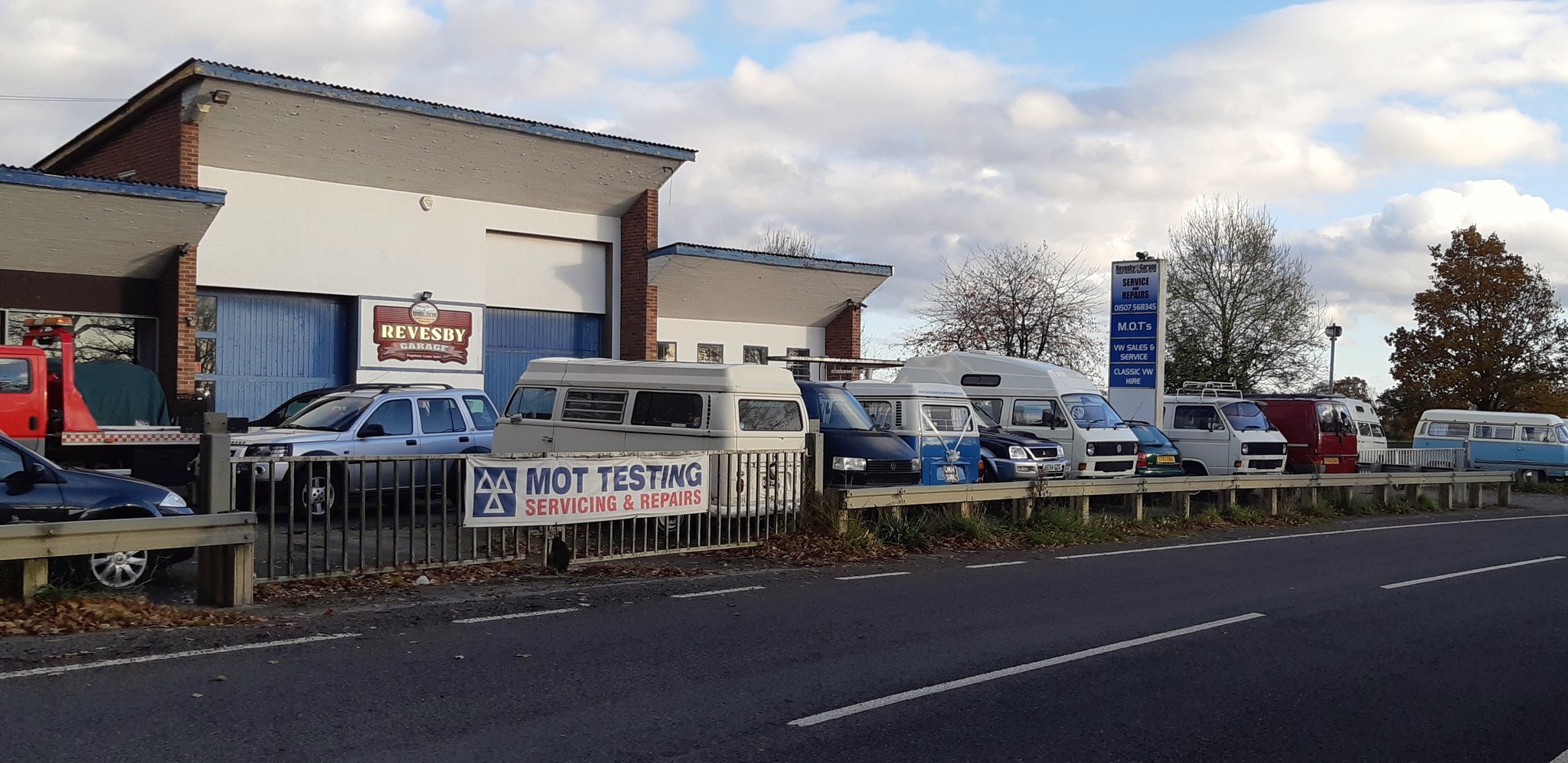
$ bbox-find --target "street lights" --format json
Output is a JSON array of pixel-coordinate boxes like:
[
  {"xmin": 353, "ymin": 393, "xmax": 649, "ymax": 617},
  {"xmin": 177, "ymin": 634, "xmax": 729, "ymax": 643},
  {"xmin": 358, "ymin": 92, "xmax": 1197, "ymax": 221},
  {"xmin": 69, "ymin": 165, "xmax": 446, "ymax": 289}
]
[{"xmin": 1325, "ymin": 323, "xmax": 1341, "ymax": 392}]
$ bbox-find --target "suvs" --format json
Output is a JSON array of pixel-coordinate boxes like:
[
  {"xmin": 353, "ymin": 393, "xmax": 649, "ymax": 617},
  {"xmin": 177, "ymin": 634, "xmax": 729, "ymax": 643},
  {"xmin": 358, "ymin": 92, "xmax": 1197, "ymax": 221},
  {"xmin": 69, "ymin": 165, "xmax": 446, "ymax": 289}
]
[
  {"xmin": 195, "ymin": 384, "xmax": 501, "ymax": 520},
  {"xmin": 969, "ymin": 400, "xmax": 1072, "ymax": 482}
]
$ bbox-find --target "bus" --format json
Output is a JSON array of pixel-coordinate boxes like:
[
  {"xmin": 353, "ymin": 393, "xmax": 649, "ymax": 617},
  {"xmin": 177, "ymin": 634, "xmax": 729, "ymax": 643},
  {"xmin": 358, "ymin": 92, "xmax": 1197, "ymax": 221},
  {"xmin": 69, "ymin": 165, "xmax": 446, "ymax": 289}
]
[
  {"xmin": 490, "ymin": 357, "xmax": 808, "ymax": 540},
  {"xmin": 822, "ymin": 380, "xmax": 985, "ymax": 486}
]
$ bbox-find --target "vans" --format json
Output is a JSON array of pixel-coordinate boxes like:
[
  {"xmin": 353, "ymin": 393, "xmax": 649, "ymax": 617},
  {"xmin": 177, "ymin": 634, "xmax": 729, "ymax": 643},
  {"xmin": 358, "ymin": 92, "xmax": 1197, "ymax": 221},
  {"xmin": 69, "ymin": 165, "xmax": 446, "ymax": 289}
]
[
  {"xmin": 794, "ymin": 379, "xmax": 925, "ymax": 487},
  {"xmin": 1164, "ymin": 380, "xmax": 1288, "ymax": 499},
  {"xmin": 1319, "ymin": 394, "xmax": 1388, "ymax": 469},
  {"xmin": 1223, "ymin": 392, "xmax": 1361, "ymax": 475},
  {"xmin": 1413, "ymin": 409, "xmax": 1568, "ymax": 488}
]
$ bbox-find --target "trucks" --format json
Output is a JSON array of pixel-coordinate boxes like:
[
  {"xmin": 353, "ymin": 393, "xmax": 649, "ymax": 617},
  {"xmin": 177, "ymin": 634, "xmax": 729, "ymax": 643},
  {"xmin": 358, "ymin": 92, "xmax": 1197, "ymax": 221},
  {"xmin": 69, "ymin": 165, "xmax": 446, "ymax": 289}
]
[
  {"xmin": 894, "ymin": 351, "xmax": 1139, "ymax": 479},
  {"xmin": 0, "ymin": 316, "xmax": 202, "ymax": 506}
]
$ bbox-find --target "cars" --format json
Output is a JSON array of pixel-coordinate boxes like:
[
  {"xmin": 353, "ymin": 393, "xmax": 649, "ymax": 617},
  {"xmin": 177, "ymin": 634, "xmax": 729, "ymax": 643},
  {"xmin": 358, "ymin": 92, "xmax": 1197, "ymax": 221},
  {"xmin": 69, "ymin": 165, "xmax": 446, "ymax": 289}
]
[
  {"xmin": 1125, "ymin": 419, "xmax": 1186, "ymax": 476},
  {"xmin": 0, "ymin": 430, "xmax": 197, "ymax": 593},
  {"xmin": 250, "ymin": 384, "xmax": 411, "ymax": 429}
]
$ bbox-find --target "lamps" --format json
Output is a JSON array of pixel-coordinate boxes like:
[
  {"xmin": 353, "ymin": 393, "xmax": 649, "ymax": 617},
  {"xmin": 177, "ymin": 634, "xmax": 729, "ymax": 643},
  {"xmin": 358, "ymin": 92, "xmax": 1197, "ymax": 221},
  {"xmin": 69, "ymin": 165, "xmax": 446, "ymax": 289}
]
[
  {"xmin": 847, "ymin": 299, "xmax": 854, "ymax": 307},
  {"xmin": 209, "ymin": 90, "xmax": 231, "ymax": 105},
  {"xmin": 421, "ymin": 291, "xmax": 432, "ymax": 300}
]
